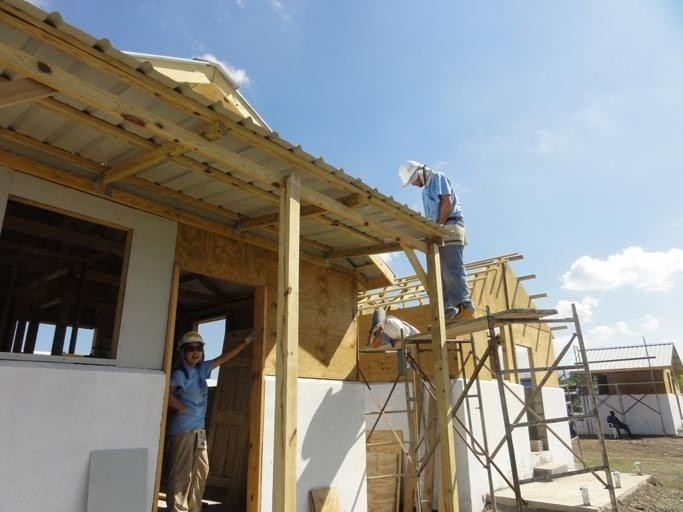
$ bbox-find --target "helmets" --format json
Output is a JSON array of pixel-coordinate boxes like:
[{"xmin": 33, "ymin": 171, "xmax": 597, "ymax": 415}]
[
  {"xmin": 176, "ymin": 331, "xmax": 204, "ymax": 354},
  {"xmin": 399, "ymin": 160, "xmax": 421, "ymax": 186}
]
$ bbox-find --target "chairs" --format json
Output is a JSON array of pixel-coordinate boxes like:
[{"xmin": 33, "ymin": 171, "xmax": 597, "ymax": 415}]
[{"xmin": 606, "ymin": 423, "xmax": 619, "ymax": 438}]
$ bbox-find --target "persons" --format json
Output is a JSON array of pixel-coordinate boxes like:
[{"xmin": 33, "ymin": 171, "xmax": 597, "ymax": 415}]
[
  {"xmin": 164, "ymin": 322, "xmax": 259, "ymax": 512},
  {"xmin": 566, "ymin": 405, "xmax": 576, "ymax": 436},
  {"xmin": 369, "ymin": 316, "xmax": 420, "ymax": 378},
  {"xmin": 396, "ymin": 157, "xmax": 476, "ymax": 331},
  {"xmin": 605, "ymin": 411, "xmax": 632, "ymax": 437}
]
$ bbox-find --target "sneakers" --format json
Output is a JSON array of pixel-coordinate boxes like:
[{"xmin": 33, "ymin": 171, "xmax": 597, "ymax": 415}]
[{"xmin": 446, "ymin": 304, "xmax": 475, "ymax": 325}]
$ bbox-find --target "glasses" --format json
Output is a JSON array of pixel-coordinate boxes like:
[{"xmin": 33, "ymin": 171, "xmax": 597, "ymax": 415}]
[{"xmin": 187, "ymin": 345, "xmax": 203, "ymax": 352}]
